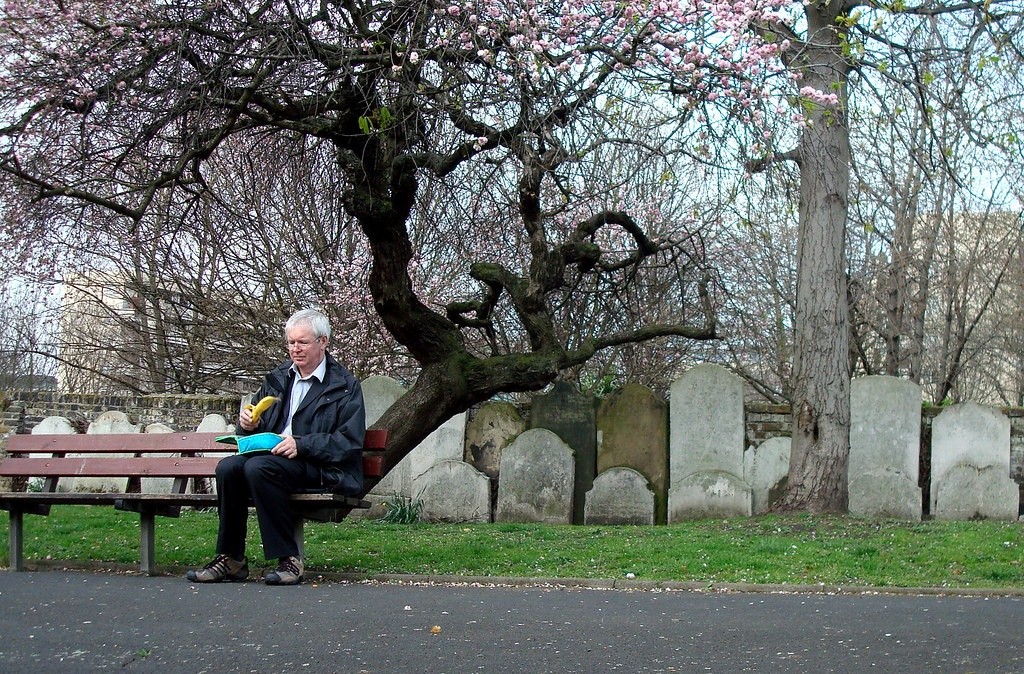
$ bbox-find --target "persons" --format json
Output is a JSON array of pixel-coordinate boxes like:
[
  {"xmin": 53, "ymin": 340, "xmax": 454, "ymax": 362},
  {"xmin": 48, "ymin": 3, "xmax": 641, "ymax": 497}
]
[{"xmin": 187, "ymin": 309, "xmax": 365, "ymax": 584}]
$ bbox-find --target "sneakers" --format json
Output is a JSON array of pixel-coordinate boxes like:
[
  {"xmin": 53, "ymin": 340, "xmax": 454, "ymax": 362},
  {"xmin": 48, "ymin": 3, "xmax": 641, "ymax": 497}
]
[
  {"xmin": 187, "ymin": 552, "xmax": 249, "ymax": 583},
  {"xmin": 266, "ymin": 556, "xmax": 304, "ymax": 585}
]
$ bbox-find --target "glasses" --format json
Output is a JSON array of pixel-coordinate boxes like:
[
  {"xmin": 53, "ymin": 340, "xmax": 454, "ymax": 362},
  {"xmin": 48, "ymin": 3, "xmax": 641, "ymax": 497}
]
[{"xmin": 285, "ymin": 335, "xmax": 322, "ymax": 351}]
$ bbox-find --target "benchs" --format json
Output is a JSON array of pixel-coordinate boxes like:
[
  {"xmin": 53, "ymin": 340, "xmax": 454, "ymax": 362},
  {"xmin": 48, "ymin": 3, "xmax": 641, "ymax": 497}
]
[{"xmin": 0, "ymin": 430, "xmax": 390, "ymax": 574}]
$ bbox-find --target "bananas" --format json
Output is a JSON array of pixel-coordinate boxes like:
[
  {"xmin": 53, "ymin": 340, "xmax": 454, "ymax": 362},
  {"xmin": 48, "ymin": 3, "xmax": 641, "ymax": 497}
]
[{"xmin": 243, "ymin": 396, "xmax": 281, "ymax": 422}]
